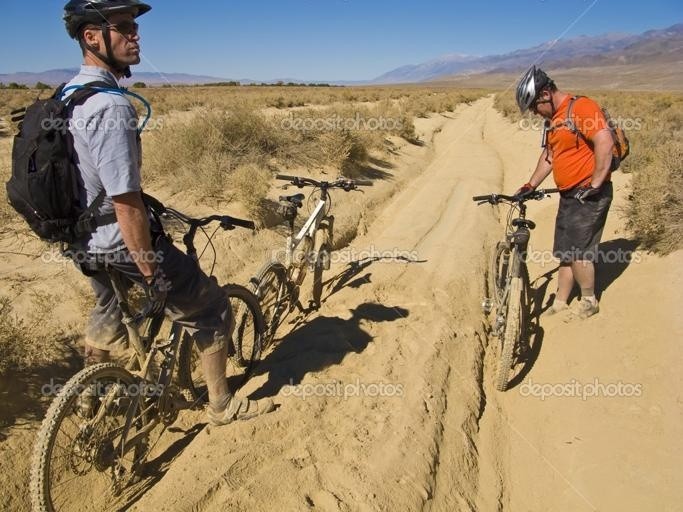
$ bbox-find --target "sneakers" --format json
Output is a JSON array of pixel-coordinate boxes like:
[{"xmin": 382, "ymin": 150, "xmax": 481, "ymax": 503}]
[
  {"xmin": 203, "ymin": 394, "xmax": 275, "ymax": 428},
  {"xmin": 561, "ymin": 296, "xmax": 599, "ymax": 326},
  {"xmin": 537, "ymin": 303, "xmax": 569, "ymax": 319},
  {"xmin": 76, "ymin": 396, "xmax": 128, "ymax": 419}
]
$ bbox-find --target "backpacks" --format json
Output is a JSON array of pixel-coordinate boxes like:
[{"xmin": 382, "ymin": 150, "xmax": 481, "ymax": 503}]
[
  {"xmin": 567, "ymin": 95, "xmax": 631, "ymax": 171},
  {"xmin": 4, "ymin": 80, "xmax": 119, "ymax": 246}
]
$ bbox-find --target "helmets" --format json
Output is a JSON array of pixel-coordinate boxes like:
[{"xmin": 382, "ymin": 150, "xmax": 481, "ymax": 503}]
[
  {"xmin": 61, "ymin": 0, "xmax": 153, "ymax": 19},
  {"xmin": 514, "ymin": 64, "xmax": 539, "ymax": 115}
]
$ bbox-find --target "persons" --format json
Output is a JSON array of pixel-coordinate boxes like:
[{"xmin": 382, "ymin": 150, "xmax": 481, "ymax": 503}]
[
  {"xmin": 56, "ymin": 0, "xmax": 274, "ymax": 425},
  {"xmin": 511, "ymin": 66, "xmax": 615, "ymax": 325}
]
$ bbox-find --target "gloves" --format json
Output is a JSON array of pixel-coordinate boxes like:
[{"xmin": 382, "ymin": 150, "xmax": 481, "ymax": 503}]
[
  {"xmin": 514, "ymin": 183, "xmax": 536, "ymax": 200},
  {"xmin": 144, "ymin": 272, "xmax": 171, "ymax": 318},
  {"xmin": 573, "ymin": 181, "xmax": 601, "ymax": 205}
]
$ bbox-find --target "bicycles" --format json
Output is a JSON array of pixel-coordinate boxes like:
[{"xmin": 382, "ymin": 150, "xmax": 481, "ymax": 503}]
[
  {"xmin": 29, "ymin": 192, "xmax": 264, "ymax": 512},
  {"xmin": 472, "ymin": 188, "xmax": 561, "ymax": 392},
  {"xmin": 229, "ymin": 174, "xmax": 373, "ymax": 366}
]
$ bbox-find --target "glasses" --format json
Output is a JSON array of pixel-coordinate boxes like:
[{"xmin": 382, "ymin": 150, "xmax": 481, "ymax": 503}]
[{"xmin": 83, "ymin": 22, "xmax": 139, "ymax": 34}]
